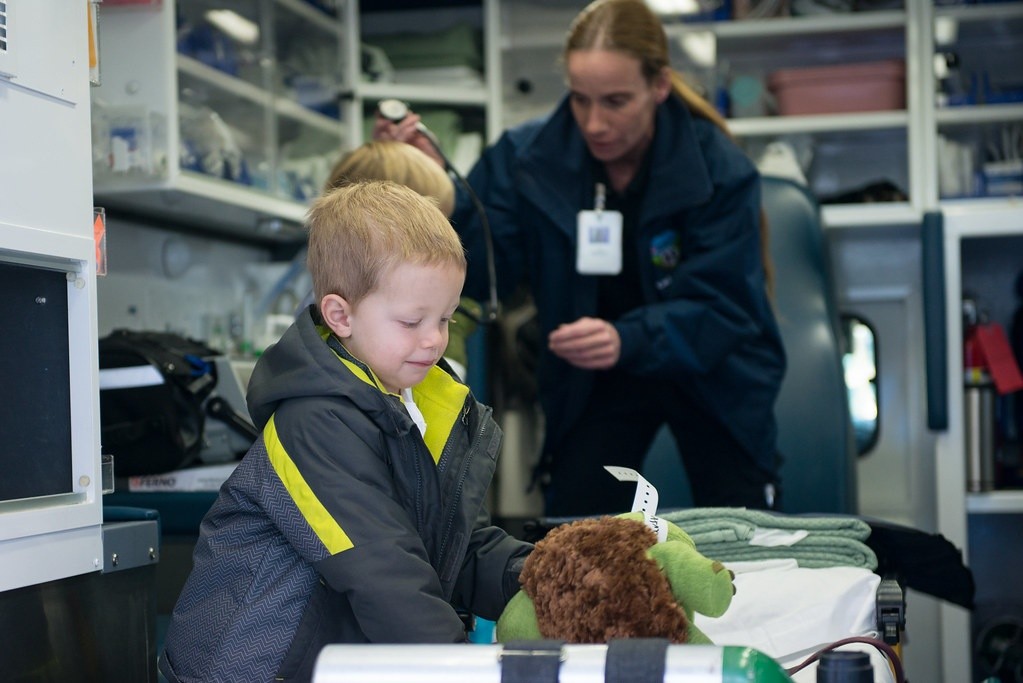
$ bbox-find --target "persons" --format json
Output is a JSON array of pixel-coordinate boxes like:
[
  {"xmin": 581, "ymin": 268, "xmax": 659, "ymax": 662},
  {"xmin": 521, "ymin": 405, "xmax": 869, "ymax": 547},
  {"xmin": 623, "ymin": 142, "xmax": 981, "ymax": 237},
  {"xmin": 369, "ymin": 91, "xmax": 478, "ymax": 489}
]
[
  {"xmin": 155, "ymin": 143, "xmax": 535, "ymax": 683},
  {"xmin": 370, "ymin": 1, "xmax": 783, "ymax": 521}
]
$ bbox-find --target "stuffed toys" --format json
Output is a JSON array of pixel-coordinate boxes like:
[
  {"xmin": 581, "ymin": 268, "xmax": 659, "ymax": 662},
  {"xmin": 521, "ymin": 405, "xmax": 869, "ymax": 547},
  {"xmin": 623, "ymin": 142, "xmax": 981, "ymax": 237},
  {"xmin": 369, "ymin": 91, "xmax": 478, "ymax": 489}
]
[{"xmin": 493, "ymin": 503, "xmax": 737, "ymax": 646}]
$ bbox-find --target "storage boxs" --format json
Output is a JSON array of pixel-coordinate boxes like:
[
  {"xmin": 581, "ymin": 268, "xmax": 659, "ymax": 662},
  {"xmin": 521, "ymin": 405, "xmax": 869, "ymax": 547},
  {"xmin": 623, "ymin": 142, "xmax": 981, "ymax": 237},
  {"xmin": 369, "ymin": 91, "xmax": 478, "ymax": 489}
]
[{"xmin": 766, "ymin": 57, "xmax": 904, "ymax": 116}]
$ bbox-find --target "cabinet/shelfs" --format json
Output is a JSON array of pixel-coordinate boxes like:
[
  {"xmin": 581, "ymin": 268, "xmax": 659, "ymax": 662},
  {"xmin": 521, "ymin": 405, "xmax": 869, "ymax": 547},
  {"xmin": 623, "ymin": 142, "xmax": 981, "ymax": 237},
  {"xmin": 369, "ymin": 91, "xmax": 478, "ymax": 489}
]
[{"xmin": 88, "ymin": 0, "xmax": 1023, "ymax": 683}]
